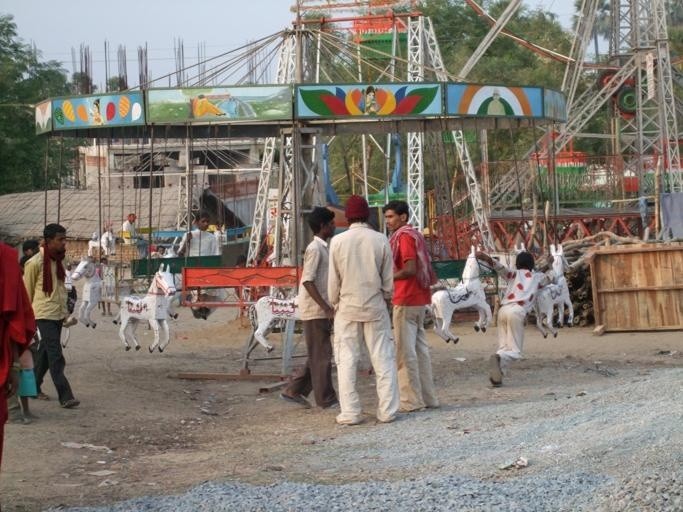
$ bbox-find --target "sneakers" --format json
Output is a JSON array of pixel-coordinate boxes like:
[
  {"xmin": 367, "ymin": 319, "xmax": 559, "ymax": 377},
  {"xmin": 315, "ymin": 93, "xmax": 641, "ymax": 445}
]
[
  {"xmin": 61, "ymin": 399, "xmax": 79, "ymax": 409},
  {"xmin": 488, "ymin": 355, "xmax": 503, "ymax": 385},
  {"xmin": 35, "ymin": 393, "xmax": 48, "ymax": 400}
]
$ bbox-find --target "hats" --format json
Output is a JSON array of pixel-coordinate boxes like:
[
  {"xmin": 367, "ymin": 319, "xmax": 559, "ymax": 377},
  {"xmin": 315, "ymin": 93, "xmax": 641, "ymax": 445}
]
[{"xmin": 344, "ymin": 195, "xmax": 370, "ymax": 220}]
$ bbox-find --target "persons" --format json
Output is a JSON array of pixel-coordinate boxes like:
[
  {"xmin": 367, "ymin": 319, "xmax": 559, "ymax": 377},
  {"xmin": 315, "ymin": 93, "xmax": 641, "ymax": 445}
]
[
  {"xmin": 23, "ymin": 223, "xmax": 80, "ymax": 408},
  {"xmin": 383, "ymin": 200, "xmax": 438, "ymax": 411},
  {"xmin": 175, "ymin": 213, "xmax": 219, "ymax": 320},
  {"xmin": 96, "ymin": 259, "xmax": 112, "ymax": 315},
  {"xmin": 474, "ymin": 251, "xmax": 557, "ymax": 388},
  {"xmin": 157, "ymin": 245, "xmax": 165, "ymax": 255},
  {"xmin": 0, "ymin": 236, "xmax": 38, "ymax": 487},
  {"xmin": 327, "ymin": 196, "xmax": 400, "ymax": 423},
  {"xmin": 120, "ymin": 212, "xmax": 150, "ymax": 259},
  {"xmin": 281, "ymin": 207, "xmax": 341, "ymax": 413}
]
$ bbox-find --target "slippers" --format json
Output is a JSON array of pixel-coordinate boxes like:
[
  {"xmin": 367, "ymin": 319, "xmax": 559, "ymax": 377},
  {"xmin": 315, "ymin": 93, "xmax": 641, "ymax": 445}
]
[
  {"xmin": 318, "ymin": 401, "xmax": 340, "ymax": 410},
  {"xmin": 281, "ymin": 391, "xmax": 308, "ymax": 406}
]
